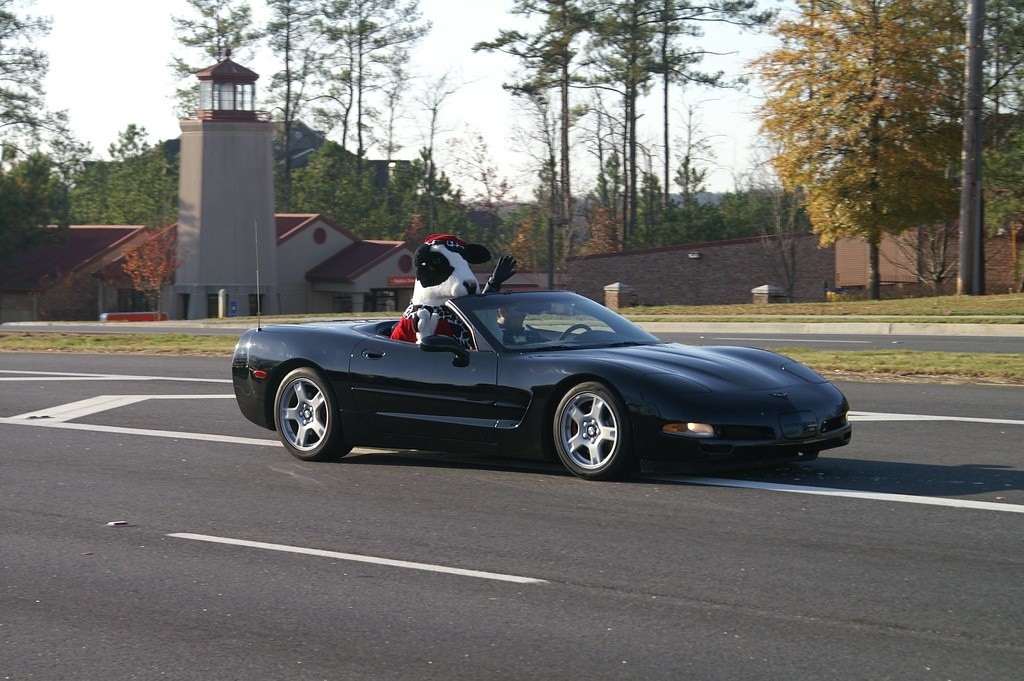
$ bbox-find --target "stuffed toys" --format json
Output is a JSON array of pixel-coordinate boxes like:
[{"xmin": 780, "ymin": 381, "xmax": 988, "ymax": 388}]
[{"xmin": 390, "ymin": 233, "xmax": 492, "ymax": 350}]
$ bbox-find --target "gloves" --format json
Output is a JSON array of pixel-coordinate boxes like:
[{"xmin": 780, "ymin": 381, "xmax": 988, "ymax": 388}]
[{"xmin": 488, "ymin": 255, "xmax": 517, "ymax": 291}]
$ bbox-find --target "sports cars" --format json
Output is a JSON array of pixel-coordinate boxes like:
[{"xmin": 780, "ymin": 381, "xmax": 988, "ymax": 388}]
[{"xmin": 233, "ymin": 288, "xmax": 853, "ymax": 481}]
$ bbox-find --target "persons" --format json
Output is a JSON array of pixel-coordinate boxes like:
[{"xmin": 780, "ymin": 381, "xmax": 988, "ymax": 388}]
[{"xmin": 482, "ymin": 255, "xmax": 557, "ymax": 345}]
